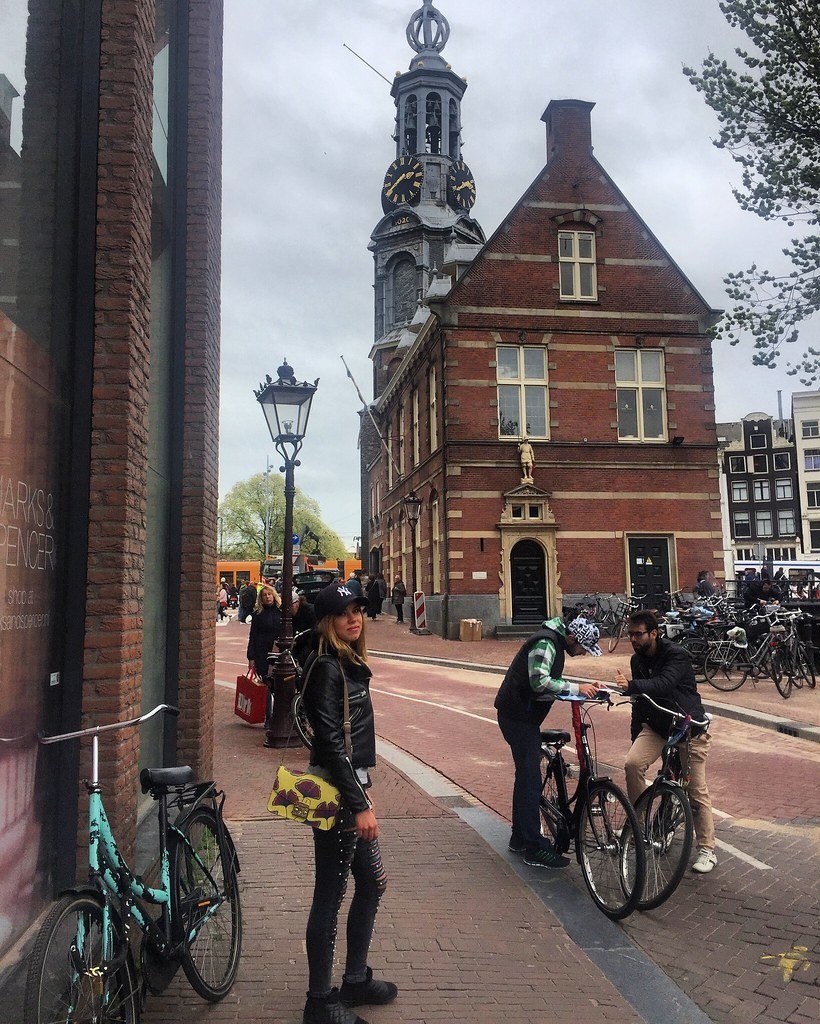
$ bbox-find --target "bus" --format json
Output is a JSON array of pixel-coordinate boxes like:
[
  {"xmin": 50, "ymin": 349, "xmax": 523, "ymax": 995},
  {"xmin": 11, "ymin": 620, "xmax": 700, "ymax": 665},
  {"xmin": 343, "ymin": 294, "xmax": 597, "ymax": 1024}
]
[
  {"xmin": 216, "ymin": 561, "xmax": 361, "ymax": 605},
  {"xmin": 734, "ymin": 560, "xmax": 820, "ymax": 598}
]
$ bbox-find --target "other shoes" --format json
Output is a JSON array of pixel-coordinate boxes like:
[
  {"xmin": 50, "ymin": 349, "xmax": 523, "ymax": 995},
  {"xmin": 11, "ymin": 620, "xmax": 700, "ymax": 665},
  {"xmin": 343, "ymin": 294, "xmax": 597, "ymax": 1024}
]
[
  {"xmin": 264, "ymin": 719, "xmax": 270, "ymax": 730},
  {"xmin": 398, "ymin": 621, "xmax": 402, "ymax": 624}
]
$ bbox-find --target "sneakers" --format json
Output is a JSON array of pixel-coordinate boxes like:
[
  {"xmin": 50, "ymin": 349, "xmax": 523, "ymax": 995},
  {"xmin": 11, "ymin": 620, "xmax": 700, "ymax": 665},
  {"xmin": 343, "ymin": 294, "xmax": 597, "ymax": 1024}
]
[
  {"xmin": 508, "ymin": 836, "xmax": 528, "ymax": 851},
  {"xmin": 339, "ymin": 967, "xmax": 397, "ymax": 1008},
  {"xmin": 523, "ymin": 846, "xmax": 572, "ymax": 869},
  {"xmin": 612, "ymin": 828, "xmax": 623, "ymax": 837},
  {"xmin": 693, "ymin": 848, "xmax": 718, "ymax": 873},
  {"xmin": 303, "ymin": 987, "xmax": 369, "ymax": 1024}
]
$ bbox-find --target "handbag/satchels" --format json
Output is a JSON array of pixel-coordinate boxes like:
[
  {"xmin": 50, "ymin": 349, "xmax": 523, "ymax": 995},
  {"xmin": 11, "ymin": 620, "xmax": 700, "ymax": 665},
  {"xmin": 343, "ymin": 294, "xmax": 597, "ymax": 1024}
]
[
  {"xmin": 221, "ymin": 602, "xmax": 226, "ymax": 607},
  {"xmin": 234, "ymin": 667, "xmax": 267, "ymax": 723},
  {"xmin": 267, "ymin": 766, "xmax": 342, "ymax": 831}
]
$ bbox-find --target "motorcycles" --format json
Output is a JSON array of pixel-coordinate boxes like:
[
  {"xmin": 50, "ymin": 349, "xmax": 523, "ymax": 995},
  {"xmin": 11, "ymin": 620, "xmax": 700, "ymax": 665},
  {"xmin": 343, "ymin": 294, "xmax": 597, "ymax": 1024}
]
[{"xmin": 229, "ymin": 594, "xmax": 238, "ymax": 609}]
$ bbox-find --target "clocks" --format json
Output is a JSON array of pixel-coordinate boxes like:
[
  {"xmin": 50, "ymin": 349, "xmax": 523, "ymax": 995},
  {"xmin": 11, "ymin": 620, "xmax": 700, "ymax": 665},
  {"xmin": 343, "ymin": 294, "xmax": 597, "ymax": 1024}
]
[
  {"xmin": 448, "ymin": 160, "xmax": 477, "ymax": 211},
  {"xmin": 383, "ymin": 154, "xmax": 425, "ymax": 206}
]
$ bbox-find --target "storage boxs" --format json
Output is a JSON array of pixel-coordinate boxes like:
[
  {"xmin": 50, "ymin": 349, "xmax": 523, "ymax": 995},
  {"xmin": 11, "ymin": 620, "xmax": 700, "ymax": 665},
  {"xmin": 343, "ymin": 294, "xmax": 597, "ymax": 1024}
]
[{"xmin": 458, "ymin": 619, "xmax": 482, "ymax": 642}]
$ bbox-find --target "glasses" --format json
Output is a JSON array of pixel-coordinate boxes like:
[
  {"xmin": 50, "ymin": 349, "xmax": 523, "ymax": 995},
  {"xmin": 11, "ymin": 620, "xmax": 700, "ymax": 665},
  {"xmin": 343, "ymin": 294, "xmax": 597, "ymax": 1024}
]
[{"xmin": 626, "ymin": 631, "xmax": 648, "ymax": 639}]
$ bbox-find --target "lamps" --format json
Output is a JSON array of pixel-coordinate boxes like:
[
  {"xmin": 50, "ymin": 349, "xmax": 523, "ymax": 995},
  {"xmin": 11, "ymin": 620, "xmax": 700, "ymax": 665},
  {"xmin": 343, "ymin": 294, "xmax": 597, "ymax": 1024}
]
[{"xmin": 672, "ymin": 434, "xmax": 685, "ymax": 444}]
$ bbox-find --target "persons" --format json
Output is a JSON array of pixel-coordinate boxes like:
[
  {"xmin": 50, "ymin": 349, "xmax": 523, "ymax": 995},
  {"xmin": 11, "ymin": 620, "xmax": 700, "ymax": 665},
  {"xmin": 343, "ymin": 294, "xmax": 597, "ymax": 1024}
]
[
  {"xmin": 696, "ymin": 570, "xmax": 716, "ymax": 600},
  {"xmin": 340, "ymin": 568, "xmax": 388, "ymax": 620},
  {"xmin": 247, "ymin": 586, "xmax": 282, "ymax": 729},
  {"xmin": 517, "ymin": 435, "xmax": 535, "ymax": 479},
  {"xmin": 613, "ymin": 611, "xmax": 718, "ymax": 872},
  {"xmin": 217, "ymin": 574, "xmax": 280, "ymax": 624},
  {"xmin": 300, "ymin": 583, "xmax": 397, "ymax": 1024},
  {"xmin": 391, "ymin": 575, "xmax": 405, "ymax": 625},
  {"xmin": 743, "ymin": 580, "xmax": 783, "ymax": 646},
  {"xmin": 279, "ymin": 591, "xmax": 321, "ymax": 720},
  {"xmin": 743, "ymin": 565, "xmax": 820, "ymax": 601},
  {"xmin": 494, "ymin": 617, "xmax": 603, "ymax": 869}
]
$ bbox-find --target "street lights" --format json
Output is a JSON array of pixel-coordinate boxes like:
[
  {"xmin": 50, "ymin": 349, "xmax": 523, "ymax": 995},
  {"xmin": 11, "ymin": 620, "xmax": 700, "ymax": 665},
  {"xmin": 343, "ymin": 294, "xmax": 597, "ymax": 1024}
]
[
  {"xmin": 402, "ymin": 490, "xmax": 424, "ymax": 633},
  {"xmin": 252, "ymin": 356, "xmax": 321, "ymax": 749}
]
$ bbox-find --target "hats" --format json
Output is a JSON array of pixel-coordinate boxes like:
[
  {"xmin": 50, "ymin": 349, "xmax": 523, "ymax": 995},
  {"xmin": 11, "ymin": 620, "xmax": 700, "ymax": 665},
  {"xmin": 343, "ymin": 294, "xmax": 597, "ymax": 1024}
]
[
  {"xmin": 292, "ymin": 590, "xmax": 299, "ymax": 603},
  {"xmin": 313, "ymin": 582, "xmax": 370, "ymax": 619},
  {"xmin": 568, "ymin": 618, "xmax": 604, "ymax": 656}
]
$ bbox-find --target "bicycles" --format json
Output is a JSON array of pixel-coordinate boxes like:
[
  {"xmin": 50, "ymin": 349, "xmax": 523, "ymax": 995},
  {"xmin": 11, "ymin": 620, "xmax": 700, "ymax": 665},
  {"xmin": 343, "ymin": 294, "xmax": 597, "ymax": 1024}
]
[
  {"xmin": 539, "ymin": 688, "xmax": 646, "ymax": 922},
  {"xmin": 267, "ymin": 628, "xmax": 315, "ymax": 751},
  {"xmin": 566, "ymin": 589, "xmax": 816, "ymax": 699},
  {"xmin": 615, "ymin": 692, "xmax": 712, "ymax": 910},
  {"xmin": 24, "ymin": 704, "xmax": 246, "ymax": 1024}
]
